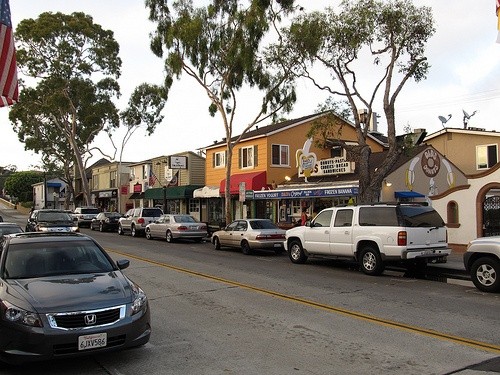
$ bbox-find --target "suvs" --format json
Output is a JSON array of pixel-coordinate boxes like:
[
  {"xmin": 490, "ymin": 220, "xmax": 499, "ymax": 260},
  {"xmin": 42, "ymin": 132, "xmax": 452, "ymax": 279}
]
[
  {"xmin": 73, "ymin": 206, "xmax": 99, "ymax": 228},
  {"xmin": 25, "ymin": 209, "xmax": 80, "ymax": 234},
  {"xmin": 117, "ymin": 207, "xmax": 164, "ymax": 236},
  {"xmin": 284, "ymin": 203, "xmax": 452, "ymax": 276}
]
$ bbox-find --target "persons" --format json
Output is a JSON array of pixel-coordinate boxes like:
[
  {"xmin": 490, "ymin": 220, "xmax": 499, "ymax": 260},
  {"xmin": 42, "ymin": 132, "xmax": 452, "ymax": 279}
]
[{"xmin": 301, "ymin": 207, "xmax": 317, "ymax": 226}]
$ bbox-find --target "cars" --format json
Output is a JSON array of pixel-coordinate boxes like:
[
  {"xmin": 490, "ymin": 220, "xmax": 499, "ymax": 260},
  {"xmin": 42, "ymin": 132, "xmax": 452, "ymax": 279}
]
[
  {"xmin": 0, "ymin": 222, "xmax": 25, "ymax": 251},
  {"xmin": 211, "ymin": 219, "xmax": 286, "ymax": 255},
  {"xmin": 145, "ymin": 213, "xmax": 208, "ymax": 243},
  {"xmin": 0, "ymin": 231, "xmax": 152, "ymax": 366},
  {"xmin": 463, "ymin": 235, "xmax": 500, "ymax": 292},
  {"xmin": 90, "ymin": 212, "xmax": 123, "ymax": 232}
]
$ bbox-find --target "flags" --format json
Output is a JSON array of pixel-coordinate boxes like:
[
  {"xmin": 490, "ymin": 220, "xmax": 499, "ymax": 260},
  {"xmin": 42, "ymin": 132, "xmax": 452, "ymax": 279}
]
[
  {"xmin": 149, "ymin": 170, "xmax": 157, "ymax": 187},
  {"xmin": 166, "ymin": 171, "xmax": 178, "ymax": 187},
  {"xmin": 0, "ymin": 0, "xmax": 20, "ymax": 107}
]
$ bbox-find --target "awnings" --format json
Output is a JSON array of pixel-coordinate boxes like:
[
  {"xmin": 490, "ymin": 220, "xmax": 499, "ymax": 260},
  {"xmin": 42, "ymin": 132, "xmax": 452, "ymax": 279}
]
[
  {"xmin": 129, "ymin": 171, "xmax": 360, "ymax": 199},
  {"xmin": 395, "ymin": 191, "xmax": 425, "ymax": 198},
  {"xmin": 92, "ymin": 189, "xmax": 117, "ymax": 199}
]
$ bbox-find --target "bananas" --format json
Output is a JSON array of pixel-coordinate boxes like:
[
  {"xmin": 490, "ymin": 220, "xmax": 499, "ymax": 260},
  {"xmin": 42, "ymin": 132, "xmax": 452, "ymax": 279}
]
[{"xmin": 295, "ymin": 140, "xmax": 317, "ymax": 177}]
